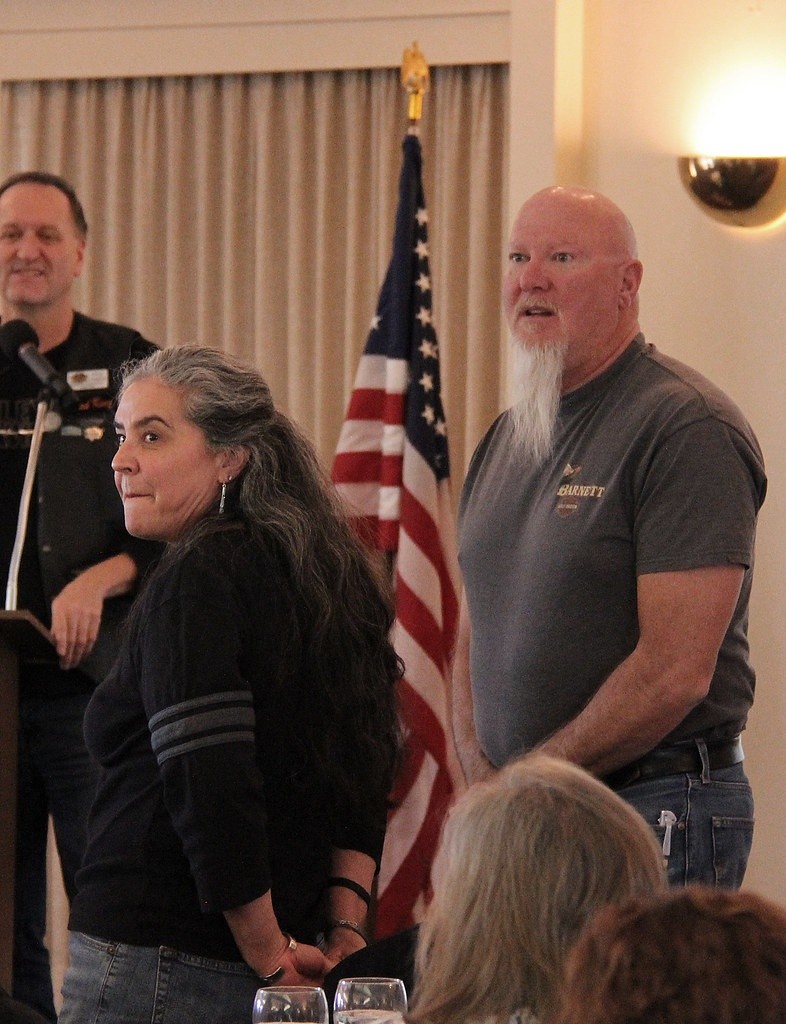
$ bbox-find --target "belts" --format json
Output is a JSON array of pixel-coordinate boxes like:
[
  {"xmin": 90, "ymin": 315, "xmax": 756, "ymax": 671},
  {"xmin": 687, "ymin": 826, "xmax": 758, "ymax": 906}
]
[{"xmin": 601, "ymin": 734, "xmax": 744, "ymax": 791}]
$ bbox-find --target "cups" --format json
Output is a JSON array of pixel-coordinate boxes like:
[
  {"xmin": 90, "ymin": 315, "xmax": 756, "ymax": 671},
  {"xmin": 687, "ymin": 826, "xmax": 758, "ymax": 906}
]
[
  {"xmin": 252, "ymin": 985, "xmax": 329, "ymax": 1024},
  {"xmin": 333, "ymin": 976, "xmax": 408, "ymax": 1024}
]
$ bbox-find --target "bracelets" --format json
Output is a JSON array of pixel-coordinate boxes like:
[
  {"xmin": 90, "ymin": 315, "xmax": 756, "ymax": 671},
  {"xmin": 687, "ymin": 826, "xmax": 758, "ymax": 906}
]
[
  {"xmin": 330, "ymin": 919, "xmax": 368, "ymax": 945},
  {"xmin": 330, "ymin": 875, "xmax": 371, "ymax": 909},
  {"xmin": 258, "ymin": 931, "xmax": 298, "ymax": 984}
]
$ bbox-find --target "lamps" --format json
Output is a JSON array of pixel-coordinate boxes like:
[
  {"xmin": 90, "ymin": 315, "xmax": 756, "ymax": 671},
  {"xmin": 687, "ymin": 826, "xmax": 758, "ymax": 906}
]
[{"xmin": 677, "ymin": 155, "xmax": 786, "ymax": 230}]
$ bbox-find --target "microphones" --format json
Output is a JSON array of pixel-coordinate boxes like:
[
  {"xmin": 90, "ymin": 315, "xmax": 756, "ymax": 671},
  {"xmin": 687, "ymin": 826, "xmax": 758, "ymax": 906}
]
[{"xmin": 0, "ymin": 319, "xmax": 80, "ymax": 410}]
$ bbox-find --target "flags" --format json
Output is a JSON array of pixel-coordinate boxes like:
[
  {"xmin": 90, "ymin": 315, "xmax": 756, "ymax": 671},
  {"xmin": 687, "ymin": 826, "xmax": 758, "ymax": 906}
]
[{"xmin": 327, "ymin": 130, "xmax": 467, "ymax": 943}]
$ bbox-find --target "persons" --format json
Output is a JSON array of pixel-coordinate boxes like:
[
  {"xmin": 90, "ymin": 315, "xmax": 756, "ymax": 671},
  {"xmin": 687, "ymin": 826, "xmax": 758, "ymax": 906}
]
[
  {"xmin": 0, "ymin": 170, "xmax": 164, "ymax": 1024},
  {"xmin": 561, "ymin": 882, "xmax": 786, "ymax": 1024},
  {"xmin": 444, "ymin": 184, "xmax": 768, "ymax": 893},
  {"xmin": 60, "ymin": 345, "xmax": 403, "ymax": 1024},
  {"xmin": 407, "ymin": 757, "xmax": 669, "ymax": 1024}
]
